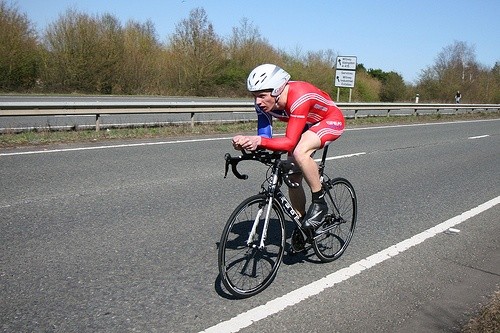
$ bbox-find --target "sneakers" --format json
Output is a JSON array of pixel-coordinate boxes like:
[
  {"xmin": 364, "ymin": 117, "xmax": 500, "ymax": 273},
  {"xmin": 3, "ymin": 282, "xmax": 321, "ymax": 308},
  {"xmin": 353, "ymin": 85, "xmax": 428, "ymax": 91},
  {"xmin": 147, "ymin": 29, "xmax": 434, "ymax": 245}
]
[{"xmin": 302, "ymin": 201, "xmax": 328, "ymax": 229}]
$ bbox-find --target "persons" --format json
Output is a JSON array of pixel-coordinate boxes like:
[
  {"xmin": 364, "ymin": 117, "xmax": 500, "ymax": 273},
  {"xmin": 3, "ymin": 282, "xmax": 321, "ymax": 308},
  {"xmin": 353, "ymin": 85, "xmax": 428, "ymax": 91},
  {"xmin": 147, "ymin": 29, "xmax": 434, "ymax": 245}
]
[
  {"xmin": 231, "ymin": 64, "xmax": 346, "ymax": 252},
  {"xmin": 455, "ymin": 91, "xmax": 461, "ymax": 103}
]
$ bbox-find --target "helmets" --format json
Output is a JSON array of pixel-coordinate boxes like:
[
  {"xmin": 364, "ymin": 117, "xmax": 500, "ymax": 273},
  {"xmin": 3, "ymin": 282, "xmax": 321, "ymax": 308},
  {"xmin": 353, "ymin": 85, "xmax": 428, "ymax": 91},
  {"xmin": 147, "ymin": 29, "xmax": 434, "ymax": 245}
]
[{"xmin": 246, "ymin": 63, "xmax": 291, "ymax": 97}]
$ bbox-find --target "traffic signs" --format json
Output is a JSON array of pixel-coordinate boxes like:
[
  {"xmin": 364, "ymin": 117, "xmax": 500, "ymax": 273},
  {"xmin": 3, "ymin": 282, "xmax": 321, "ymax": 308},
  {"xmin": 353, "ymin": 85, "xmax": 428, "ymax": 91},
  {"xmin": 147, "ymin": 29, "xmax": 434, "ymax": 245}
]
[
  {"xmin": 334, "ymin": 70, "xmax": 356, "ymax": 88},
  {"xmin": 336, "ymin": 55, "xmax": 357, "ymax": 71}
]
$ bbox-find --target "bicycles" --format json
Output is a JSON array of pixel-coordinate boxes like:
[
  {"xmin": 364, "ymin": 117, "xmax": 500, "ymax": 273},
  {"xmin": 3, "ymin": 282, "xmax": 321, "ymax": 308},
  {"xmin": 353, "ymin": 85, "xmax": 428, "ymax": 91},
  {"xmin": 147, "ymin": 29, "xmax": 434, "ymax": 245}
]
[{"xmin": 217, "ymin": 139, "xmax": 360, "ymax": 300}]
